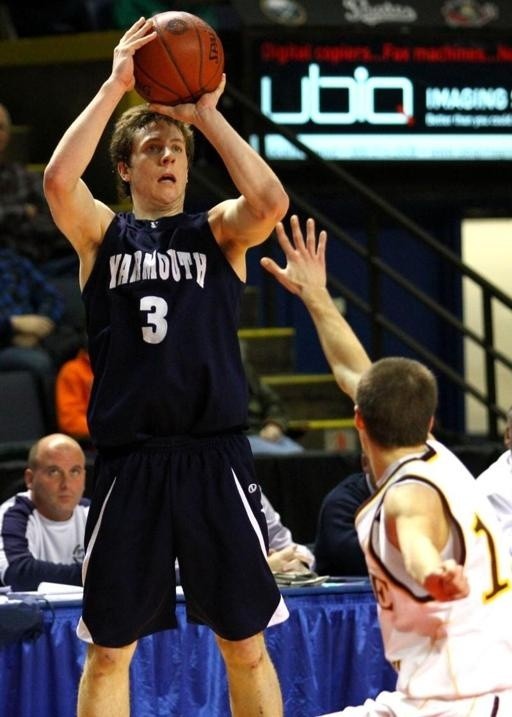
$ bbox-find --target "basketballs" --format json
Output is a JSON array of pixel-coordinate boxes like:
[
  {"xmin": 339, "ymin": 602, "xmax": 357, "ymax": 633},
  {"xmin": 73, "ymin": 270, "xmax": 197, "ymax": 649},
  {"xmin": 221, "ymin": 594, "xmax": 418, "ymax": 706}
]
[{"xmin": 134, "ymin": 11, "xmax": 225, "ymax": 105}]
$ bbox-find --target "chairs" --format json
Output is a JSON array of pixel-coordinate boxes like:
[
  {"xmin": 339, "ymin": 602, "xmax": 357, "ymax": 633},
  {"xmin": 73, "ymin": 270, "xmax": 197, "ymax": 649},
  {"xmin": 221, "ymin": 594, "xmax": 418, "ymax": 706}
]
[{"xmin": 0, "ymin": 370, "xmax": 44, "ymax": 444}]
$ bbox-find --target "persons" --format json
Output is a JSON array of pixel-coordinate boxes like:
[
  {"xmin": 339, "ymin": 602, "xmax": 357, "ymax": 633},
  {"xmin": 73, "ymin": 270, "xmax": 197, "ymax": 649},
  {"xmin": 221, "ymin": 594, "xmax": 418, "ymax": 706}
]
[
  {"xmin": 0, "ymin": 430, "xmax": 98, "ymax": 592},
  {"xmin": 52, "ymin": 306, "xmax": 96, "ymax": 444},
  {"xmin": 478, "ymin": 410, "xmax": 512, "ymax": 550},
  {"xmin": 312, "ymin": 438, "xmax": 381, "ymax": 579},
  {"xmin": 36, "ymin": 8, "xmax": 305, "ymax": 716},
  {"xmin": 258, "ymin": 483, "xmax": 320, "ymax": 577},
  {"xmin": 259, "ymin": 211, "xmax": 512, "ymax": 717},
  {"xmin": 0, "ymin": 97, "xmax": 62, "ymax": 268},
  {"xmin": 230, "ymin": 354, "xmax": 287, "ymax": 459},
  {"xmin": 1, "ymin": 239, "xmax": 71, "ymax": 423}
]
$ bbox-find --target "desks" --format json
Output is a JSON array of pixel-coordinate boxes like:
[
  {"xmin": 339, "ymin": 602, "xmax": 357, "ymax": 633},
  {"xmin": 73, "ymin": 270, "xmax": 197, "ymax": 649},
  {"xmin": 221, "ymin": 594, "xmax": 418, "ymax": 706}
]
[{"xmin": 0, "ymin": 577, "xmax": 398, "ymax": 717}]
